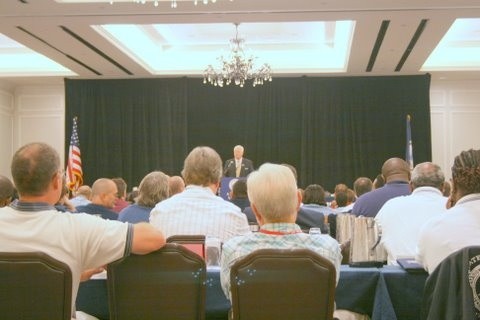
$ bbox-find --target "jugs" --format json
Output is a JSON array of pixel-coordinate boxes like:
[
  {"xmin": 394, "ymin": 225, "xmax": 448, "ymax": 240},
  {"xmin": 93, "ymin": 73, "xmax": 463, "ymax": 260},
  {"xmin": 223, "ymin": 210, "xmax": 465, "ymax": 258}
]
[
  {"xmin": 336, "ymin": 212, "xmax": 355, "ymax": 244},
  {"xmin": 350, "ymin": 215, "xmax": 382, "ymax": 266}
]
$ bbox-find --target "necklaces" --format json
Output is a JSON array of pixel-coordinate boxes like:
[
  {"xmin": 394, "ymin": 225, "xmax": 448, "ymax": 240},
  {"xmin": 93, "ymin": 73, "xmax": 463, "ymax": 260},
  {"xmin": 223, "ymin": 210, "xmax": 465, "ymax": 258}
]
[{"xmin": 259, "ymin": 228, "xmax": 301, "ymax": 235}]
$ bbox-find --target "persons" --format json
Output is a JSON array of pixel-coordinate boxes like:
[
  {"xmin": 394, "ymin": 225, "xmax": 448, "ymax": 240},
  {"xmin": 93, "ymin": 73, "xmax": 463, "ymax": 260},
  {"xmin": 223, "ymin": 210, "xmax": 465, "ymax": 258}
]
[{"xmin": 0, "ymin": 144, "xmax": 480, "ymax": 320}]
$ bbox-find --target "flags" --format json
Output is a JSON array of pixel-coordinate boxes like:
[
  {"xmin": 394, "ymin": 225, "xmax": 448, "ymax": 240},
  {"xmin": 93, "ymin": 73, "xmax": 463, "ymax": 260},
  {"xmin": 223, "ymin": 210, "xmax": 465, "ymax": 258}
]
[
  {"xmin": 406, "ymin": 116, "xmax": 414, "ymax": 171},
  {"xmin": 63, "ymin": 119, "xmax": 83, "ymax": 199}
]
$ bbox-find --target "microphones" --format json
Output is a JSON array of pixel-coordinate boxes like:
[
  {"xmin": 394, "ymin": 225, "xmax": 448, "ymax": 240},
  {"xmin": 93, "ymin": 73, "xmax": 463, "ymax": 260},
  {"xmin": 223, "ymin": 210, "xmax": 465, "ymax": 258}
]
[{"xmin": 228, "ymin": 161, "xmax": 233, "ymax": 167}]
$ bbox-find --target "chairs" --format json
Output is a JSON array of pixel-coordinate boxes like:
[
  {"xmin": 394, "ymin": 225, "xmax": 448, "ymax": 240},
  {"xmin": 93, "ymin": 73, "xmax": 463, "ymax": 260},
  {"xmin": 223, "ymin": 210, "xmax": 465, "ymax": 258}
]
[
  {"xmin": 0, "ymin": 251, "xmax": 72, "ymax": 320},
  {"xmin": 107, "ymin": 242, "xmax": 206, "ymax": 320},
  {"xmin": 421, "ymin": 246, "xmax": 480, "ymax": 320},
  {"xmin": 229, "ymin": 247, "xmax": 336, "ymax": 320}
]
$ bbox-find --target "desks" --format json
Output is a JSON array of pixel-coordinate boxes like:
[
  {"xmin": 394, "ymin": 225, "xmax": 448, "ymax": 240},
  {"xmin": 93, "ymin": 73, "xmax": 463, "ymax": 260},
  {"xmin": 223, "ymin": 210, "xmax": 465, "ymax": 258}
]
[{"xmin": 76, "ymin": 264, "xmax": 428, "ymax": 320}]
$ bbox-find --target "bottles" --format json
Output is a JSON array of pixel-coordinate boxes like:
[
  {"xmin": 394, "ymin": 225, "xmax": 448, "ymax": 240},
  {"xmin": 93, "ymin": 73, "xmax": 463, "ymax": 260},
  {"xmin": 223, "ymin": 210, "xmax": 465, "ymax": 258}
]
[{"xmin": 310, "ymin": 228, "xmax": 321, "ymax": 235}]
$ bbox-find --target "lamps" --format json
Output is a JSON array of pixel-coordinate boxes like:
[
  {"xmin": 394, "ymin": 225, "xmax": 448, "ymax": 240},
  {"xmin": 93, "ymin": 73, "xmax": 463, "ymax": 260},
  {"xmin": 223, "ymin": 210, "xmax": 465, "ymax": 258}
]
[{"xmin": 202, "ymin": 22, "xmax": 273, "ymax": 87}]
